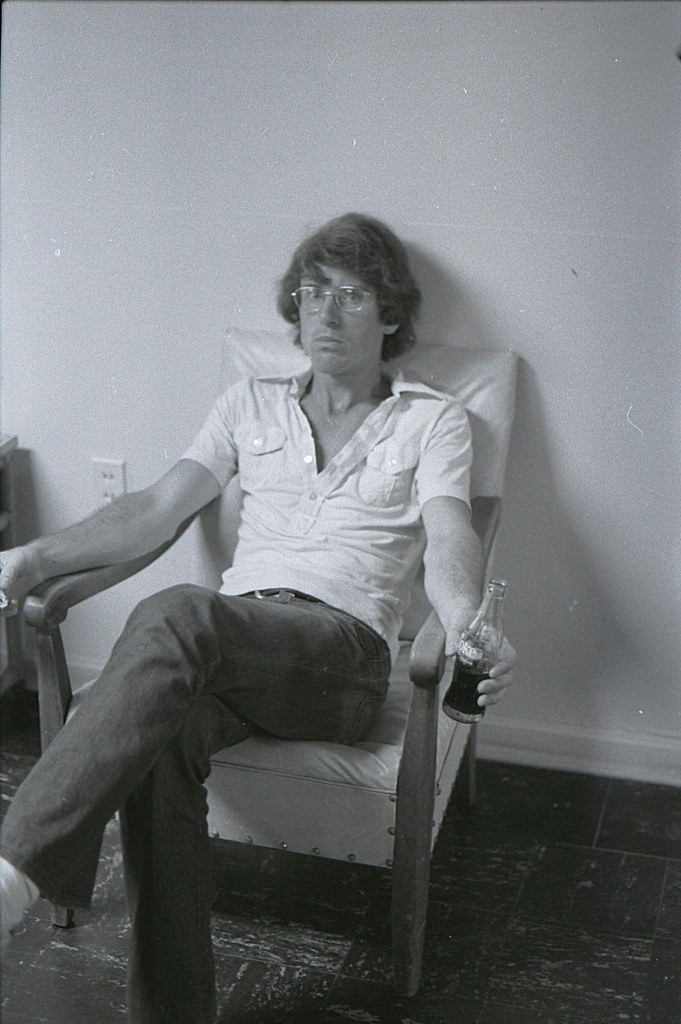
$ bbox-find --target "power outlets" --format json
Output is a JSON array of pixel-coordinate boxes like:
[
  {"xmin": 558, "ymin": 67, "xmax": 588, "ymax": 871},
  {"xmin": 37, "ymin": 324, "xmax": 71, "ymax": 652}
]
[{"xmin": 91, "ymin": 458, "xmax": 127, "ymax": 510}]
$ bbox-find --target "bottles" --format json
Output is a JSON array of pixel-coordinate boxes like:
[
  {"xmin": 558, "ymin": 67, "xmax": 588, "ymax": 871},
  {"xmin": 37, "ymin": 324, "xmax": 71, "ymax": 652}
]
[{"xmin": 442, "ymin": 577, "xmax": 509, "ymax": 723}]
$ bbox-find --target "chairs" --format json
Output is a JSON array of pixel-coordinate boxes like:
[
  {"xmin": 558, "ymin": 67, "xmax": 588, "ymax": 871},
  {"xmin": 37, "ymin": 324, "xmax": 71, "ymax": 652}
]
[
  {"xmin": 31, "ymin": 330, "xmax": 523, "ymax": 998},
  {"xmin": 0, "ymin": 433, "xmax": 26, "ymax": 695}
]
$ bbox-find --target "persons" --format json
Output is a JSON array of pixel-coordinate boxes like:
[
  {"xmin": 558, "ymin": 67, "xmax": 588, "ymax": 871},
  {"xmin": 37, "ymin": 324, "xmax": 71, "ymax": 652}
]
[{"xmin": 0, "ymin": 212, "xmax": 516, "ymax": 1024}]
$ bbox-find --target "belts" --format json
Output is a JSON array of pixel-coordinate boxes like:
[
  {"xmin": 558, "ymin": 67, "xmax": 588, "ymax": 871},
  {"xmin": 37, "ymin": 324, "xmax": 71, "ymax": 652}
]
[{"xmin": 237, "ymin": 587, "xmax": 326, "ymax": 604}]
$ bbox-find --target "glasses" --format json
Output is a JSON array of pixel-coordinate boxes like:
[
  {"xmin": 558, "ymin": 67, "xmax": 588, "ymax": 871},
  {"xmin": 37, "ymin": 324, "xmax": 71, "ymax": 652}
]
[{"xmin": 290, "ymin": 285, "xmax": 383, "ymax": 313}]
